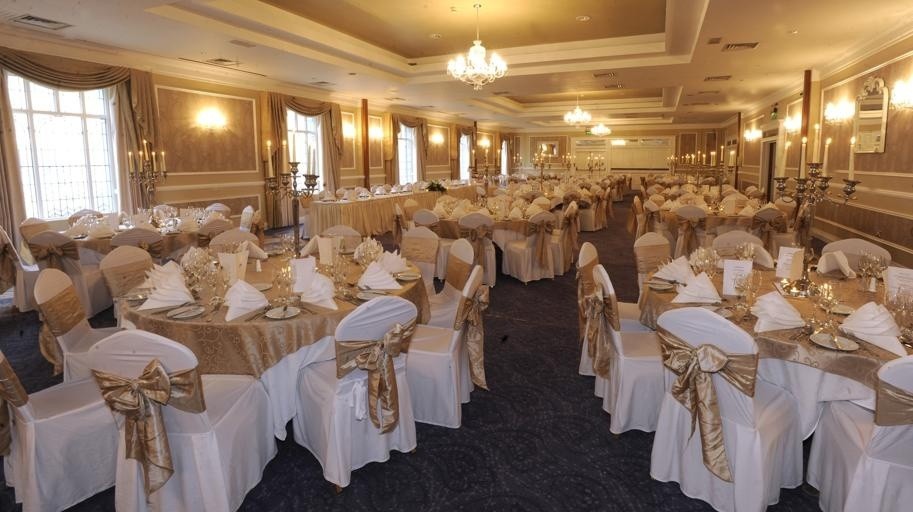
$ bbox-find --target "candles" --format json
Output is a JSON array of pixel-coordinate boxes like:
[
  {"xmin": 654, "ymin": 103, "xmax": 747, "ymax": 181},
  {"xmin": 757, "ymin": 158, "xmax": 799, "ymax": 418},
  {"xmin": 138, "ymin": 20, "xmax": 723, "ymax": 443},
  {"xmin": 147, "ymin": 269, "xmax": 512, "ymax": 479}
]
[
  {"xmin": 266, "ymin": 135, "xmax": 315, "ymax": 177},
  {"xmin": 472, "ymin": 149, "xmax": 606, "ymax": 172},
  {"xmin": 667, "ymin": 145, "xmax": 736, "ymax": 167},
  {"xmin": 127, "ymin": 139, "xmax": 166, "ymax": 172},
  {"xmin": 779, "ymin": 123, "xmax": 856, "ymax": 181}
]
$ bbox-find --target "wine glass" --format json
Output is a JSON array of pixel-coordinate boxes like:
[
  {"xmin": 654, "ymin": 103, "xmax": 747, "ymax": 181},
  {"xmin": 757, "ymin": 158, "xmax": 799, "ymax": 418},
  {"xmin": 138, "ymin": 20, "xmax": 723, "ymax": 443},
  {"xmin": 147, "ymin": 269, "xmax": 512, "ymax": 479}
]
[
  {"xmin": 435, "ymin": 191, "xmax": 560, "ymax": 242},
  {"xmin": 119, "ymin": 227, "xmax": 402, "ymax": 325},
  {"xmin": 640, "ymin": 181, "xmax": 766, "ymax": 236},
  {"xmin": 66, "ymin": 205, "xmax": 241, "ymax": 253},
  {"xmin": 676, "ymin": 237, "xmax": 913, "ymax": 372}
]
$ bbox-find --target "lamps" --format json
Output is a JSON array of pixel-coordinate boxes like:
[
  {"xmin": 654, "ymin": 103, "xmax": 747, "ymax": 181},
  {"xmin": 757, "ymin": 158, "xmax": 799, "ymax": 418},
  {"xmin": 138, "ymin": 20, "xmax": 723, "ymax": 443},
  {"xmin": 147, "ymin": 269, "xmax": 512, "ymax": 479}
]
[
  {"xmin": 563, "ymin": 94, "xmax": 592, "ymax": 124},
  {"xmin": 446, "ymin": 3, "xmax": 509, "ymax": 91},
  {"xmin": 591, "ymin": 123, "xmax": 611, "ymax": 137}
]
